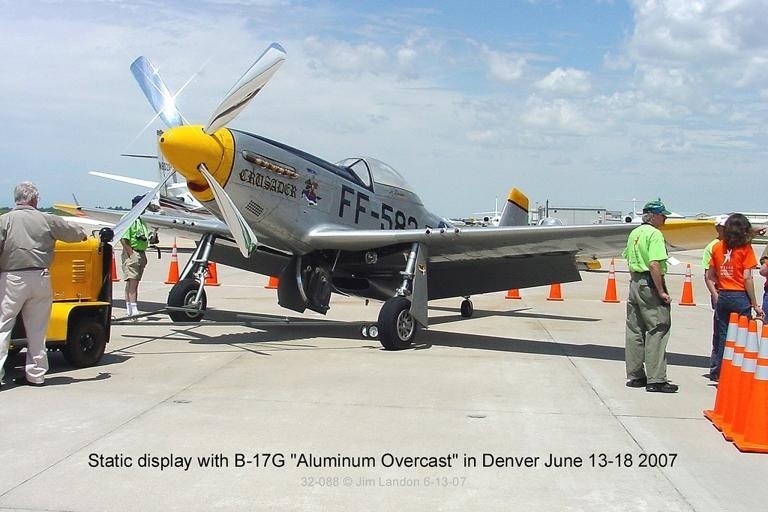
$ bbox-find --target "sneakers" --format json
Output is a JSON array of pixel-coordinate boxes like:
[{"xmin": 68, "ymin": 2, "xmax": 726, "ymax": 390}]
[
  {"xmin": 130, "ymin": 316, "xmax": 148, "ymax": 321},
  {"xmin": 626, "ymin": 376, "xmax": 647, "ymax": 387},
  {"xmin": 646, "ymin": 382, "xmax": 678, "ymax": 393},
  {"xmin": 14, "ymin": 376, "xmax": 46, "ymax": 386}
]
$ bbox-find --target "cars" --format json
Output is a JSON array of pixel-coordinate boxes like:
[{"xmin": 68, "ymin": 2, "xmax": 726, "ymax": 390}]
[{"xmin": 0, "ymin": 227, "xmax": 114, "ymax": 369}]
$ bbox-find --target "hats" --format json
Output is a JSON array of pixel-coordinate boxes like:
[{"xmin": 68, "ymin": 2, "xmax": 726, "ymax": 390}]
[
  {"xmin": 714, "ymin": 214, "xmax": 729, "ymax": 226},
  {"xmin": 132, "ymin": 195, "xmax": 146, "ymax": 203},
  {"xmin": 761, "ymin": 245, "xmax": 768, "ymax": 258},
  {"xmin": 643, "ymin": 201, "xmax": 672, "ymax": 216}
]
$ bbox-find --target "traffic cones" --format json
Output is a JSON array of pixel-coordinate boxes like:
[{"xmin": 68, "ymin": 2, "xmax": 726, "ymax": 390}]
[
  {"xmin": 264, "ymin": 276, "xmax": 278, "ymax": 289},
  {"xmin": 504, "ymin": 288, "xmax": 522, "ymax": 299},
  {"xmin": 678, "ymin": 263, "xmax": 696, "ymax": 306},
  {"xmin": 111, "ymin": 250, "xmax": 121, "ymax": 281},
  {"xmin": 702, "ymin": 312, "xmax": 767, "ymax": 454},
  {"xmin": 203, "ymin": 261, "xmax": 221, "ymax": 286},
  {"xmin": 600, "ymin": 258, "xmax": 621, "ymax": 302},
  {"xmin": 164, "ymin": 236, "xmax": 181, "ymax": 284},
  {"xmin": 546, "ymin": 284, "xmax": 564, "ymax": 301}
]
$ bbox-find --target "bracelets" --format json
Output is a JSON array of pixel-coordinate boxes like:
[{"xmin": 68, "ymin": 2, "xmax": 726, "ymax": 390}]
[{"xmin": 752, "ymin": 304, "xmax": 761, "ymax": 308}]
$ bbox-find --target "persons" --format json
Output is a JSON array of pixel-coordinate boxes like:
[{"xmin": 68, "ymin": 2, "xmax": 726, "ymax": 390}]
[
  {"xmin": 708, "ymin": 213, "xmax": 765, "ymax": 384},
  {"xmin": 759, "ymin": 243, "xmax": 768, "ymax": 323},
  {"xmin": 1, "ymin": 180, "xmax": 88, "ymax": 384},
  {"xmin": 621, "ymin": 200, "xmax": 679, "ymax": 393},
  {"xmin": 701, "ymin": 215, "xmax": 731, "ymax": 307},
  {"xmin": 118, "ymin": 196, "xmax": 152, "ymax": 321}
]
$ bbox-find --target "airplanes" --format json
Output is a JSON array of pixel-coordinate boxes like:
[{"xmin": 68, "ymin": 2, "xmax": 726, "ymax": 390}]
[
  {"xmin": 716, "ymin": 212, "xmax": 768, "ymax": 226},
  {"xmin": 608, "ymin": 197, "xmax": 646, "ymax": 223},
  {"xmin": 471, "ymin": 195, "xmax": 502, "ymax": 227}
]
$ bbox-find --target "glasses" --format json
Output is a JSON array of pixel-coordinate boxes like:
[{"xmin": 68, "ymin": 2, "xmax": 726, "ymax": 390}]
[{"xmin": 36, "ymin": 196, "xmax": 40, "ymax": 201}]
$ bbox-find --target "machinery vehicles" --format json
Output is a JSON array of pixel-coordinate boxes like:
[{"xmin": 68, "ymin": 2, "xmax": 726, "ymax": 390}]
[{"xmin": 51, "ymin": 41, "xmax": 723, "ymax": 351}]
